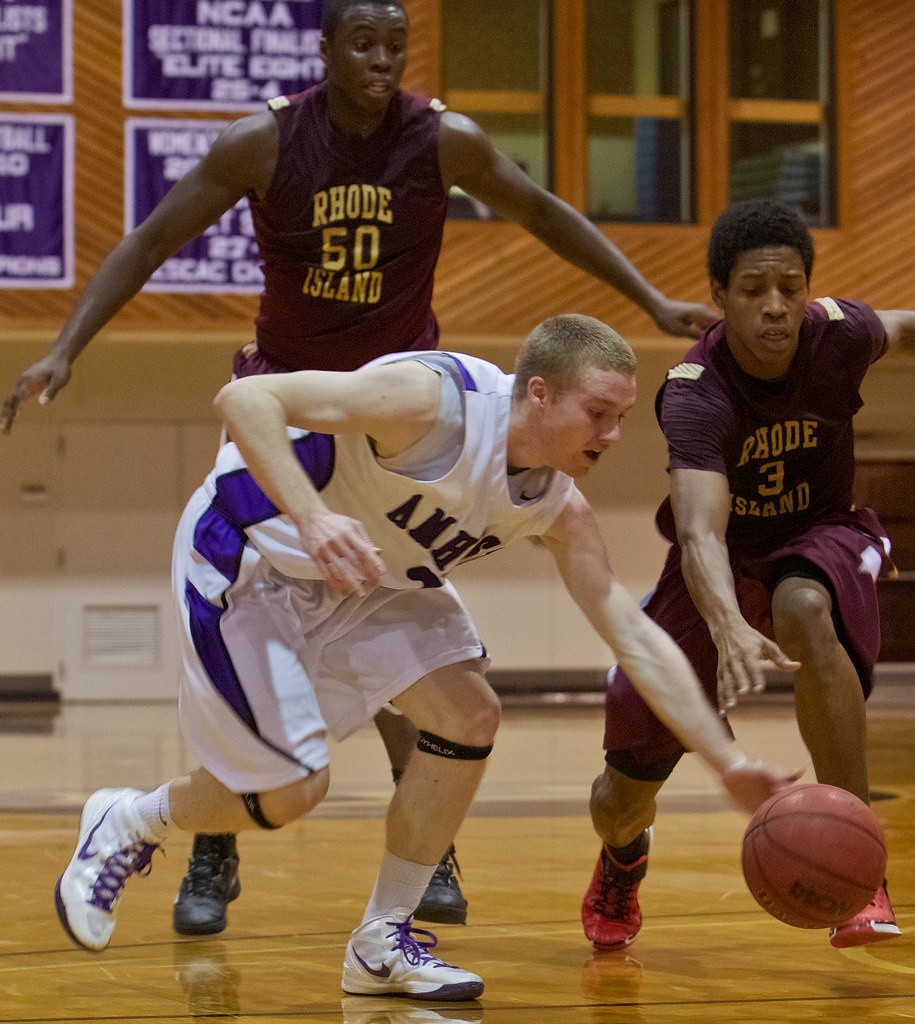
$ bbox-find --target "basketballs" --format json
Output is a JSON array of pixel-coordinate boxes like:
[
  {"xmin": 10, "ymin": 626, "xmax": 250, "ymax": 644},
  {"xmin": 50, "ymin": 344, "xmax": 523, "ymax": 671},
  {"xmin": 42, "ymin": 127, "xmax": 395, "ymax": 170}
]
[{"xmin": 739, "ymin": 781, "xmax": 890, "ymax": 930}]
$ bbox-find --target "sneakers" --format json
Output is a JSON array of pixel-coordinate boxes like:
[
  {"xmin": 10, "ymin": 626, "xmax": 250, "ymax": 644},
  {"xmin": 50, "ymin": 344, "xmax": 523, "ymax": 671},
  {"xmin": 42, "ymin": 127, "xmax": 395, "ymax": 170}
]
[
  {"xmin": 581, "ymin": 835, "xmax": 650, "ymax": 952},
  {"xmin": 174, "ymin": 841, "xmax": 241, "ymax": 936},
  {"xmin": 341, "ymin": 909, "xmax": 483, "ymax": 1002},
  {"xmin": 53, "ymin": 787, "xmax": 168, "ymax": 953},
  {"xmin": 829, "ymin": 876, "xmax": 902, "ymax": 948},
  {"xmin": 413, "ymin": 837, "xmax": 467, "ymax": 926}
]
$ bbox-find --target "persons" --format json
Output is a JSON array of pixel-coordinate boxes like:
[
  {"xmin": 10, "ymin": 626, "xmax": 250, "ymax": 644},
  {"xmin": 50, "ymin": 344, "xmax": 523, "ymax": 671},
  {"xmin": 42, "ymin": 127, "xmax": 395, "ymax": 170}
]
[
  {"xmin": 1, "ymin": 0, "xmax": 722, "ymax": 936},
  {"xmin": 161, "ymin": 935, "xmax": 644, "ymax": 1023},
  {"xmin": 580, "ymin": 203, "xmax": 915, "ymax": 954},
  {"xmin": 55, "ymin": 314, "xmax": 808, "ymax": 1003}
]
